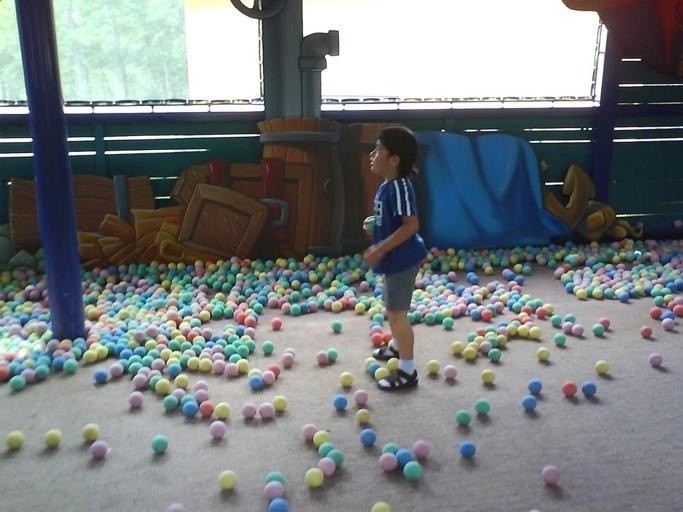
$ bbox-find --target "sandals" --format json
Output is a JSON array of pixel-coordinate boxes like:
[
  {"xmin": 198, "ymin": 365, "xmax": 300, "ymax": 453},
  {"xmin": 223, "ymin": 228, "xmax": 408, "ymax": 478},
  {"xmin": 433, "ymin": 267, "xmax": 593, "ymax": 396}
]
[{"xmin": 372, "ymin": 346, "xmax": 419, "ymax": 391}]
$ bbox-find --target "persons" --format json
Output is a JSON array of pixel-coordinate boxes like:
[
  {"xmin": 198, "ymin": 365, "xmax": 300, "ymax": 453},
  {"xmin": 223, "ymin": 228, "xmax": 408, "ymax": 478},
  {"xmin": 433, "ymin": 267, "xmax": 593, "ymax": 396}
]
[{"xmin": 355, "ymin": 125, "xmax": 427, "ymax": 390}]
